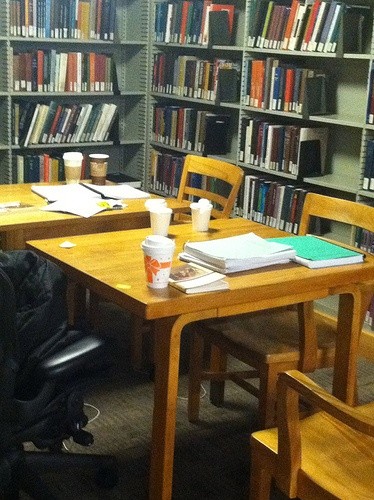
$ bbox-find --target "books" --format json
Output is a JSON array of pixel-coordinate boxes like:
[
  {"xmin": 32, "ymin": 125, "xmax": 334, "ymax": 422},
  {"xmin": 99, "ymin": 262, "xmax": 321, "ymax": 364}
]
[
  {"xmin": 12, "ymin": 153, "xmax": 91, "ymax": 184},
  {"xmin": 149, "ymin": 149, "xmax": 330, "ymax": 237},
  {"xmin": 167, "ymin": 262, "xmax": 230, "ymax": 295},
  {"xmin": 178, "ymin": 231, "xmax": 297, "ymax": 275},
  {"xmin": 355, "ymin": 226, "xmax": 374, "ymax": 253},
  {"xmin": 155, "ymin": 0, "xmax": 235, "ymax": 46},
  {"xmin": 238, "ymin": 114, "xmax": 329, "ymax": 178},
  {"xmin": 13, "ymin": 49, "xmax": 116, "ymax": 92},
  {"xmin": 245, "ymin": 56, "xmax": 329, "ymax": 115},
  {"xmin": 30, "ymin": 183, "xmax": 150, "ymax": 203},
  {"xmin": 362, "ymin": 139, "xmax": 374, "ymax": 192},
  {"xmin": 153, "ymin": 106, "xmax": 230, "ymax": 155},
  {"xmin": 265, "ymin": 235, "xmax": 365, "ymax": 269},
  {"xmin": 246, "ymin": 0, "xmax": 370, "ymax": 55},
  {"xmin": 7, "ymin": 0, "xmax": 118, "ymax": 40},
  {"xmin": 12, "ymin": 99, "xmax": 118, "ymax": 148},
  {"xmin": 153, "ymin": 51, "xmax": 241, "ymax": 103}
]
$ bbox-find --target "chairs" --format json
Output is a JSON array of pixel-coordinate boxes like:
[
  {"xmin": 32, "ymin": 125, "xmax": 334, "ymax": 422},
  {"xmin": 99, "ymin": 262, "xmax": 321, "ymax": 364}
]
[
  {"xmin": 184, "ymin": 192, "xmax": 374, "ymax": 500},
  {"xmin": 86, "ymin": 154, "xmax": 244, "ymax": 379}
]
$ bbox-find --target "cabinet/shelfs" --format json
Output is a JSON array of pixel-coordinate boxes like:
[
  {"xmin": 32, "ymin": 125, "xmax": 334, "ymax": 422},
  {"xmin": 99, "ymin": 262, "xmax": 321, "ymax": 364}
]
[
  {"xmin": 145, "ymin": 0, "xmax": 374, "ymax": 363},
  {"xmin": 0, "ymin": 0, "xmax": 151, "ymax": 192}
]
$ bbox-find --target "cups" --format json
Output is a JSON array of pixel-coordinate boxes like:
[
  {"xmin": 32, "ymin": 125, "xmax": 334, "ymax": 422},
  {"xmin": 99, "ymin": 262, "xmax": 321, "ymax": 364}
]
[
  {"xmin": 89, "ymin": 154, "xmax": 110, "ymax": 185},
  {"xmin": 150, "ymin": 207, "xmax": 173, "ymax": 237},
  {"xmin": 189, "ymin": 202, "xmax": 213, "ymax": 231},
  {"xmin": 140, "ymin": 235, "xmax": 175, "ymax": 288},
  {"xmin": 63, "ymin": 152, "xmax": 84, "ymax": 185}
]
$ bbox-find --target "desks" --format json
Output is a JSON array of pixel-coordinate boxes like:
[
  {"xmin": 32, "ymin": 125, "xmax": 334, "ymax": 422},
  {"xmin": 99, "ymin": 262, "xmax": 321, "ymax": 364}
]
[
  {"xmin": 0, "ymin": 179, "xmax": 192, "ymax": 339},
  {"xmin": 24, "ymin": 217, "xmax": 374, "ymax": 500}
]
